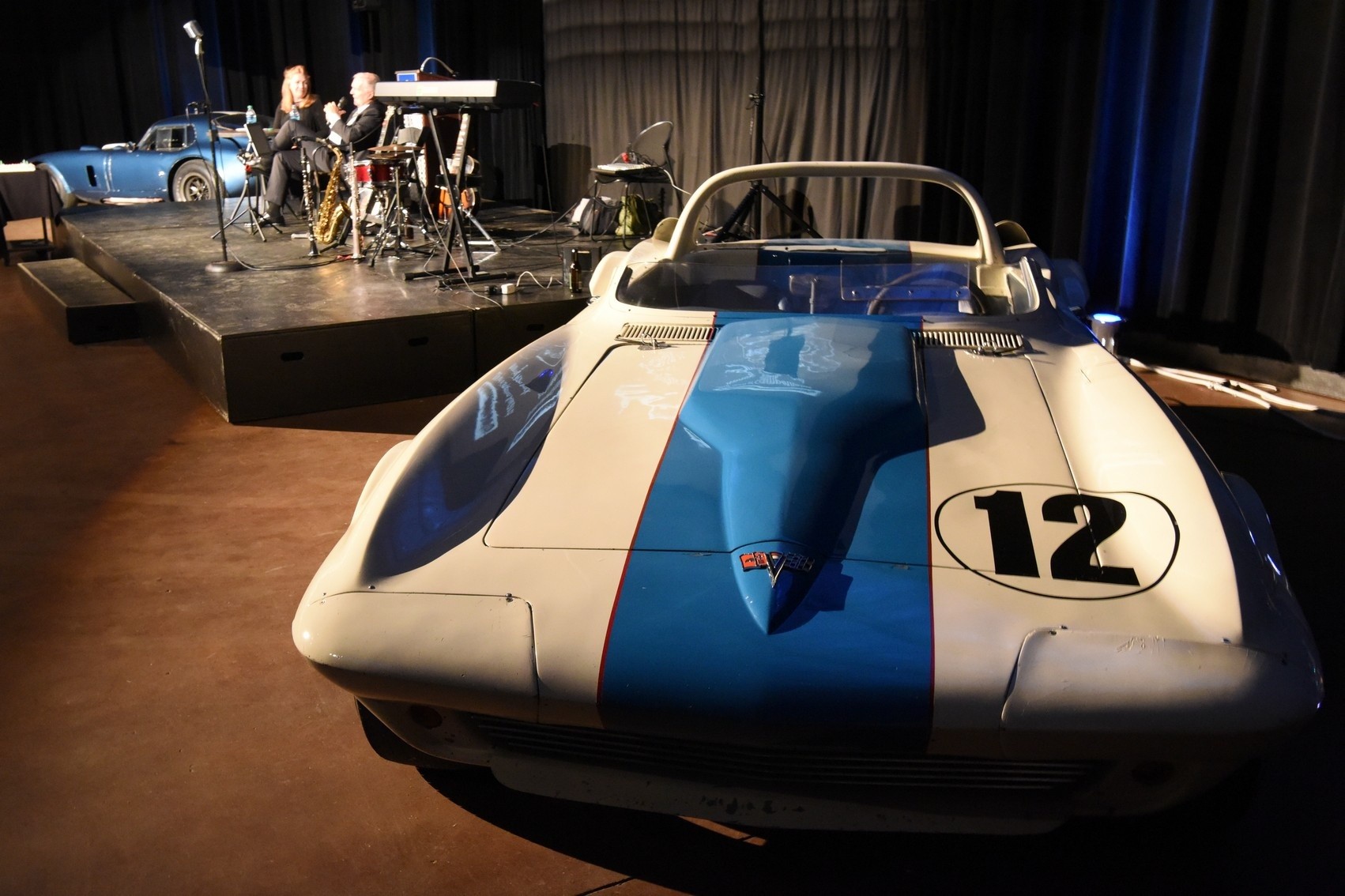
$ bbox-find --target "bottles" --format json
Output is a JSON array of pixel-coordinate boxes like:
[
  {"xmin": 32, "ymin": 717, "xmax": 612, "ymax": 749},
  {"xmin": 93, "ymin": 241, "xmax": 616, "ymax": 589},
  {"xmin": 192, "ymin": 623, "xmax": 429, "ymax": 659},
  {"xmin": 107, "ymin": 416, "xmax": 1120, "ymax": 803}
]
[
  {"xmin": 290, "ymin": 104, "xmax": 300, "ymax": 120},
  {"xmin": 246, "ymin": 105, "xmax": 257, "ymax": 123}
]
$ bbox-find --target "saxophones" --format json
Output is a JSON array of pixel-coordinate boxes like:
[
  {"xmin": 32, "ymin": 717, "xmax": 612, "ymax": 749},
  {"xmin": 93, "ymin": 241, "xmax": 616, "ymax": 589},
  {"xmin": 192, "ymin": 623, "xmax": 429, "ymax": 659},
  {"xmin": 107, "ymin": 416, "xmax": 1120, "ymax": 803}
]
[{"xmin": 295, "ymin": 135, "xmax": 353, "ymax": 245}]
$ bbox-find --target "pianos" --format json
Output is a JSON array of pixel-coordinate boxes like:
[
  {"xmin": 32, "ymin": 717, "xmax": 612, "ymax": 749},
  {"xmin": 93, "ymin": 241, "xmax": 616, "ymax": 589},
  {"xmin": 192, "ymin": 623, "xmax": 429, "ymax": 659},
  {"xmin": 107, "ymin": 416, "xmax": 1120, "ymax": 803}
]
[{"xmin": 373, "ymin": 77, "xmax": 538, "ymax": 107}]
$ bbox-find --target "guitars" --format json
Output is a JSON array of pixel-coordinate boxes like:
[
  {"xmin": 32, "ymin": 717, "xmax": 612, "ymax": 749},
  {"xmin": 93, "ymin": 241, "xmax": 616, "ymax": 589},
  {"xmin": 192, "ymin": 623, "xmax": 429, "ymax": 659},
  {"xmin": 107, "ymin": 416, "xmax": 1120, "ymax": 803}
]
[{"xmin": 436, "ymin": 110, "xmax": 482, "ymax": 223}]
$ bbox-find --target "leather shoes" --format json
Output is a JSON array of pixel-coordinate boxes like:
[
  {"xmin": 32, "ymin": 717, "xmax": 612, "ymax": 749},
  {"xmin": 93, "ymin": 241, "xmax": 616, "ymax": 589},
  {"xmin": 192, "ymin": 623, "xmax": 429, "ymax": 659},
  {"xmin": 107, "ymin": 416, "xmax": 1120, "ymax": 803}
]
[{"xmin": 244, "ymin": 214, "xmax": 285, "ymax": 228}]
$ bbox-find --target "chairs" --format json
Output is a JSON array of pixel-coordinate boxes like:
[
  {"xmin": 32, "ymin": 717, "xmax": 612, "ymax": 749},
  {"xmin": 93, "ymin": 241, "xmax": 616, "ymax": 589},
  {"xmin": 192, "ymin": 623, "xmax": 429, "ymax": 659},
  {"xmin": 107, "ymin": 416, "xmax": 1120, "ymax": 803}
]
[
  {"xmin": 697, "ymin": 278, "xmax": 795, "ymax": 313},
  {"xmin": 589, "ymin": 121, "xmax": 686, "ymax": 250},
  {"xmin": 336, "ymin": 125, "xmax": 430, "ymax": 246}
]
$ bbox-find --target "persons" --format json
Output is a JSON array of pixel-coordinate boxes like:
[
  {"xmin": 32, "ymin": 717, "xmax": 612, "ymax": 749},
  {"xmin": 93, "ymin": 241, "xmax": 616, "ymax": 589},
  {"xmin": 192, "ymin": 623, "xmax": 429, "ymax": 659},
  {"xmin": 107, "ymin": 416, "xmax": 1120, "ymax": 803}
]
[
  {"xmin": 236, "ymin": 72, "xmax": 383, "ymax": 229},
  {"xmin": 270, "ymin": 64, "xmax": 331, "ymax": 140}
]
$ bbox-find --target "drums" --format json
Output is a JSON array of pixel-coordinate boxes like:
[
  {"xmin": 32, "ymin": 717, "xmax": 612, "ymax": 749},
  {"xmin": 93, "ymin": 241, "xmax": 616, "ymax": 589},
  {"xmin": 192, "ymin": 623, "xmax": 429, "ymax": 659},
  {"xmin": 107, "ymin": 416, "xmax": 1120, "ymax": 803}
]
[{"xmin": 353, "ymin": 159, "xmax": 407, "ymax": 186}]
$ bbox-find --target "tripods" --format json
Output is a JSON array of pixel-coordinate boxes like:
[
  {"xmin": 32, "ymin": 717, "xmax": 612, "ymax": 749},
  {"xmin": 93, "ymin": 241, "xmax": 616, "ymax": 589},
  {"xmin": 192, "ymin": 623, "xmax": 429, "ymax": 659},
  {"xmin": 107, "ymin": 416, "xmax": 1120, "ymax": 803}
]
[
  {"xmin": 431, "ymin": 111, "xmax": 504, "ymax": 253},
  {"xmin": 211, "ymin": 179, "xmax": 285, "ymax": 244},
  {"xmin": 362, "ymin": 167, "xmax": 412, "ymax": 267}
]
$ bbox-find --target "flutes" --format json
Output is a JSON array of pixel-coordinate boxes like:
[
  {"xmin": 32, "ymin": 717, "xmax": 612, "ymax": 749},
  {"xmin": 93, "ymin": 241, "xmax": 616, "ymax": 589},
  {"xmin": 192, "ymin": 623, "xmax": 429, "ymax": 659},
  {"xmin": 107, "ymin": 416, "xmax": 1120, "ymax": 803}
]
[
  {"xmin": 297, "ymin": 139, "xmax": 322, "ymax": 257},
  {"xmin": 348, "ymin": 144, "xmax": 366, "ymax": 259}
]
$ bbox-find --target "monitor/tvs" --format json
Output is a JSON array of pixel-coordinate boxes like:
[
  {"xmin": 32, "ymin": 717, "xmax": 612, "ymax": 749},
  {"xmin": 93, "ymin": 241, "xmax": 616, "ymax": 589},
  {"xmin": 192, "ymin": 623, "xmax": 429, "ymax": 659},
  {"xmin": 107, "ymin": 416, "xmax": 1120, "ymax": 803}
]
[{"xmin": 244, "ymin": 122, "xmax": 273, "ymax": 157}]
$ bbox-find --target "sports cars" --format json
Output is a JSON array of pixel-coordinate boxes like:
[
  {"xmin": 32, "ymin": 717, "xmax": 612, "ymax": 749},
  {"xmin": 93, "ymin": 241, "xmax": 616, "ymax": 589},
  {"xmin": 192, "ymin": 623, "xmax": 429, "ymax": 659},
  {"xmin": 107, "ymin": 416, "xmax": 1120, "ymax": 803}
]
[
  {"xmin": 288, "ymin": 161, "xmax": 1328, "ymax": 834},
  {"xmin": 28, "ymin": 110, "xmax": 278, "ymax": 209}
]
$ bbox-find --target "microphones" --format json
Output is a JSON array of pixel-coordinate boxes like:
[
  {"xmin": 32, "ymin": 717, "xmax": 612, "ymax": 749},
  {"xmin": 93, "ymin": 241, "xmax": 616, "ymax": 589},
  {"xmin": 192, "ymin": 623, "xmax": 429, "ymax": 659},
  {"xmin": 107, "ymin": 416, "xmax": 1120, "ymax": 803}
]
[
  {"xmin": 419, "ymin": 57, "xmax": 460, "ymax": 78},
  {"xmin": 183, "ymin": 18, "xmax": 203, "ymax": 41},
  {"xmin": 325, "ymin": 97, "xmax": 346, "ymax": 125}
]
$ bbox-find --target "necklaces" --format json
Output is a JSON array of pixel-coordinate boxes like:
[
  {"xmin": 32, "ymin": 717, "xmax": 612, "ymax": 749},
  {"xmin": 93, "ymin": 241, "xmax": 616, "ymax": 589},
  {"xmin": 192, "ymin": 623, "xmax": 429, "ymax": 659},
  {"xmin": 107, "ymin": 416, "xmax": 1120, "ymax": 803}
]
[{"xmin": 291, "ymin": 100, "xmax": 301, "ymax": 105}]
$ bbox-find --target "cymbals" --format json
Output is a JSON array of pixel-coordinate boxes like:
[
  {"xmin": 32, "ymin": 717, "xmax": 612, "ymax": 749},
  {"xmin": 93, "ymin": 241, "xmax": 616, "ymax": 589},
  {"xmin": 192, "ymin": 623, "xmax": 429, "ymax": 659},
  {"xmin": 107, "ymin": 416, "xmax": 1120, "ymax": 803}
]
[
  {"xmin": 368, "ymin": 142, "xmax": 423, "ymax": 152},
  {"xmin": 366, "ymin": 154, "xmax": 425, "ymax": 158}
]
